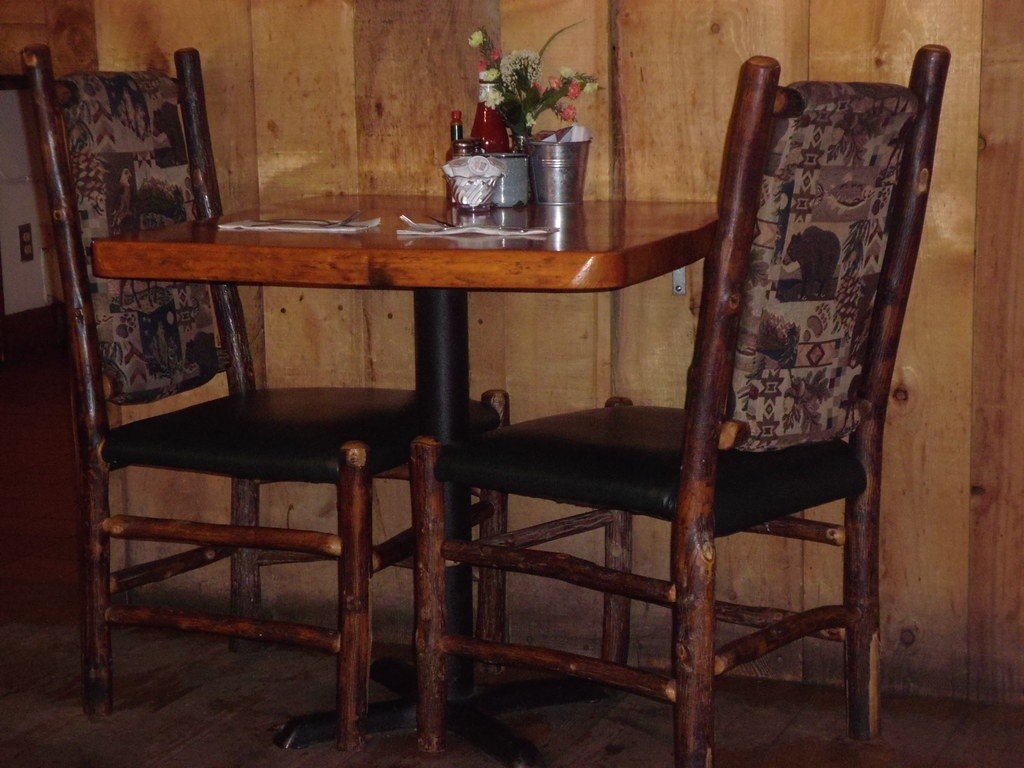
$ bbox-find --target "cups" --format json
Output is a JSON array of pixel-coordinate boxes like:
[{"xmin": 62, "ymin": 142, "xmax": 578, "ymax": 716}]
[
  {"xmin": 529, "ymin": 137, "xmax": 592, "ymax": 206},
  {"xmin": 485, "ymin": 152, "xmax": 529, "ymax": 208},
  {"xmin": 442, "ymin": 174, "xmax": 505, "ymax": 211}
]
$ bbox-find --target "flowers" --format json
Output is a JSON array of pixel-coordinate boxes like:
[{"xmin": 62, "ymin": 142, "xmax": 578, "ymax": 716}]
[{"xmin": 466, "ymin": 15, "xmax": 607, "ymax": 135}]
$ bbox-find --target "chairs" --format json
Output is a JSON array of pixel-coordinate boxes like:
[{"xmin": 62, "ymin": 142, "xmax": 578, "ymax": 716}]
[
  {"xmin": 19, "ymin": 42, "xmax": 510, "ymax": 753},
  {"xmin": 411, "ymin": 41, "xmax": 950, "ymax": 768}
]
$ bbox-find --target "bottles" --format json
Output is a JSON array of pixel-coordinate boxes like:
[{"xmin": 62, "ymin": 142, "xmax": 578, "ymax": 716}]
[
  {"xmin": 469, "ymin": 69, "xmax": 510, "ymax": 155},
  {"xmin": 445, "ymin": 109, "xmax": 483, "ymax": 209}
]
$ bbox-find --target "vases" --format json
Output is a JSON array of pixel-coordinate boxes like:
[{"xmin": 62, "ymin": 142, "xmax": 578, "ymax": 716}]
[{"xmin": 509, "ymin": 134, "xmax": 531, "ymax": 199}]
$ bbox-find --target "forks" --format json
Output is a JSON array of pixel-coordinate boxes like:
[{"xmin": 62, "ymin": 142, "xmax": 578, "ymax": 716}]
[
  {"xmin": 398, "ymin": 214, "xmax": 528, "ymax": 236},
  {"xmin": 250, "ymin": 209, "xmax": 366, "ymax": 228}
]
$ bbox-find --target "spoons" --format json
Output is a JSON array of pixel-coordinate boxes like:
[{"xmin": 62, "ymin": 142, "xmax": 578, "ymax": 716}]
[{"xmin": 422, "ymin": 211, "xmax": 525, "ymax": 231}]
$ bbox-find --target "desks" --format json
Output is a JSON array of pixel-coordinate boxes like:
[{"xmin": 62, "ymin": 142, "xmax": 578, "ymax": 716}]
[{"xmin": 90, "ymin": 191, "xmax": 715, "ymax": 768}]
